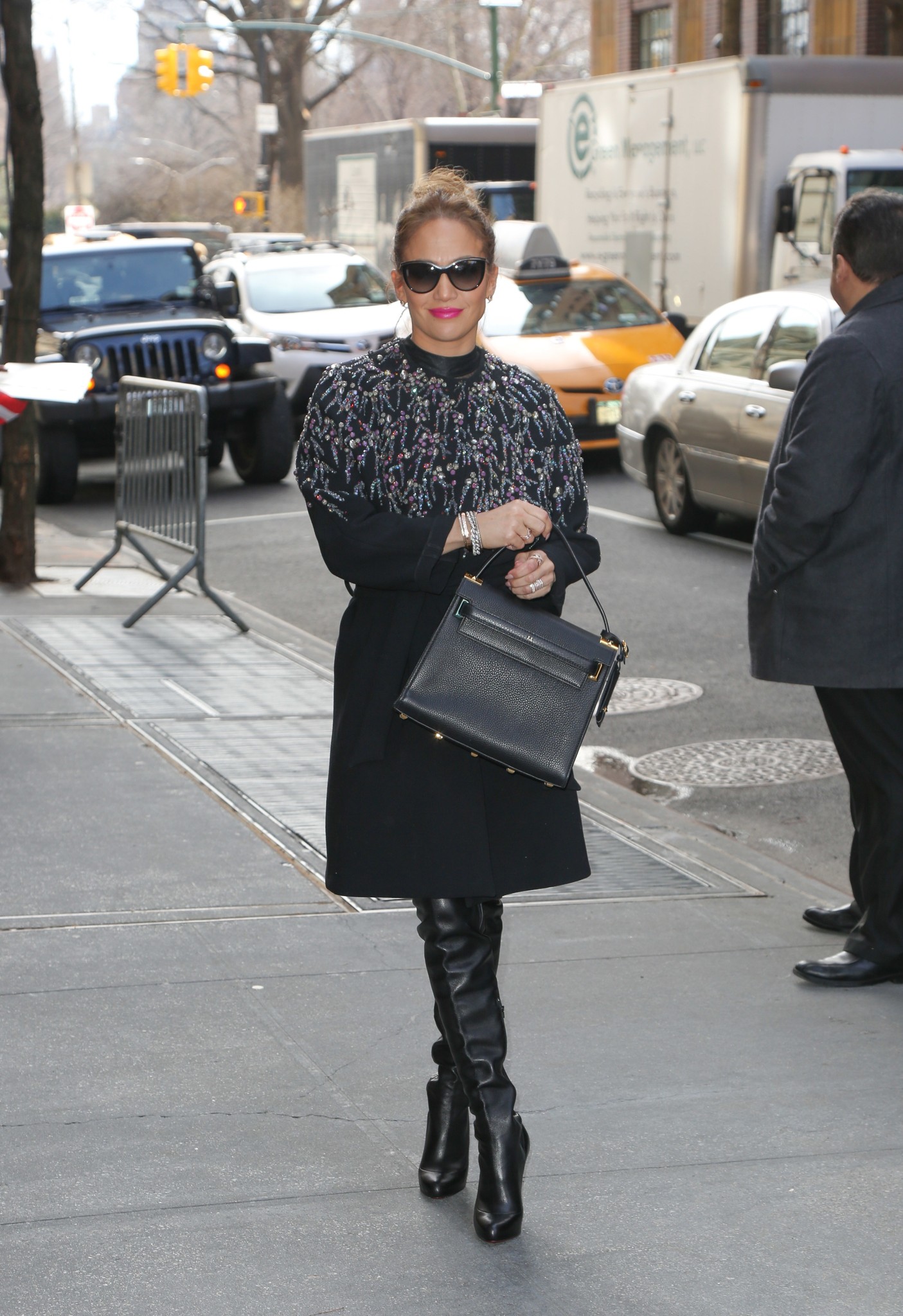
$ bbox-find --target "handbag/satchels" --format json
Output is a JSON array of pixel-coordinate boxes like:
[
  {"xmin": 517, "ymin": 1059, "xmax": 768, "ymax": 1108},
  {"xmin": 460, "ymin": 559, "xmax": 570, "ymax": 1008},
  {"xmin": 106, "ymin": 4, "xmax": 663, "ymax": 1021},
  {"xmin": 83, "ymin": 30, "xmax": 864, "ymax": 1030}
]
[{"xmin": 392, "ymin": 520, "xmax": 630, "ymax": 791}]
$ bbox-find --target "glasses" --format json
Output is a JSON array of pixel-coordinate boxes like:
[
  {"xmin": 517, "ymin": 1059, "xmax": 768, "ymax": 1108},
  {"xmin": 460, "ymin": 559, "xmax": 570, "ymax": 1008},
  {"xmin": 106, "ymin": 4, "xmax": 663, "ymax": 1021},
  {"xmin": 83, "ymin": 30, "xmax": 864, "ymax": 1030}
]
[{"xmin": 398, "ymin": 257, "xmax": 490, "ymax": 294}]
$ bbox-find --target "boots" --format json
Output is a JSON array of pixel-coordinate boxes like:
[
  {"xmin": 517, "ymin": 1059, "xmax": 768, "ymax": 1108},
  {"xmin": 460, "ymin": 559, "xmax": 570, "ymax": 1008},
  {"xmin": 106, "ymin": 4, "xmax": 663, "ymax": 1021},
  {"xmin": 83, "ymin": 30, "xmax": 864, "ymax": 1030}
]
[
  {"xmin": 413, "ymin": 897, "xmax": 531, "ymax": 1242},
  {"xmin": 418, "ymin": 897, "xmax": 504, "ymax": 1198}
]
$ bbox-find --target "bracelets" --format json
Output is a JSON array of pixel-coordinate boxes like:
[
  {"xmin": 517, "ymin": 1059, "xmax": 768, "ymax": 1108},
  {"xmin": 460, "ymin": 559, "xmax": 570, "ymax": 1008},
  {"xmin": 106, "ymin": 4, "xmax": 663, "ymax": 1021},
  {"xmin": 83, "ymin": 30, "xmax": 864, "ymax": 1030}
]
[{"xmin": 458, "ymin": 511, "xmax": 483, "ymax": 559}]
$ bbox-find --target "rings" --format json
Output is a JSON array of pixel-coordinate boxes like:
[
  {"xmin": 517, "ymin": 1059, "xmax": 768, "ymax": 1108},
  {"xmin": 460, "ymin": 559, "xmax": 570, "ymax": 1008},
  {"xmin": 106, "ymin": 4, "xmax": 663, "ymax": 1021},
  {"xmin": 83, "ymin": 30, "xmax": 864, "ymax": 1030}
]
[
  {"xmin": 520, "ymin": 528, "xmax": 531, "ymax": 540},
  {"xmin": 530, "ymin": 579, "xmax": 544, "ymax": 593},
  {"xmin": 529, "ymin": 553, "xmax": 543, "ymax": 567}
]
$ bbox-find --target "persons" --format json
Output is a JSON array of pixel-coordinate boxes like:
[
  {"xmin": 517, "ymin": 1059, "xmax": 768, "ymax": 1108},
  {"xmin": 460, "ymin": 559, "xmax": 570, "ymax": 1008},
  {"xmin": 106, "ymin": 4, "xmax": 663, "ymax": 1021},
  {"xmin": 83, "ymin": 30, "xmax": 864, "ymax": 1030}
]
[
  {"xmin": 296, "ymin": 168, "xmax": 601, "ymax": 1242},
  {"xmin": 747, "ymin": 190, "xmax": 903, "ymax": 987}
]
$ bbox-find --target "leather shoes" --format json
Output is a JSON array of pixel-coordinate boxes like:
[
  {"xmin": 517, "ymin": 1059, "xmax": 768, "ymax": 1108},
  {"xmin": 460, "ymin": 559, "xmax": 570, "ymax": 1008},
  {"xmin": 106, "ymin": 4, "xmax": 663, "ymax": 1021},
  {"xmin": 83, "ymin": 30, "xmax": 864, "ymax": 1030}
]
[
  {"xmin": 793, "ymin": 950, "xmax": 903, "ymax": 989},
  {"xmin": 802, "ymin": 902, "xmax": 859, "ymax": 930}
]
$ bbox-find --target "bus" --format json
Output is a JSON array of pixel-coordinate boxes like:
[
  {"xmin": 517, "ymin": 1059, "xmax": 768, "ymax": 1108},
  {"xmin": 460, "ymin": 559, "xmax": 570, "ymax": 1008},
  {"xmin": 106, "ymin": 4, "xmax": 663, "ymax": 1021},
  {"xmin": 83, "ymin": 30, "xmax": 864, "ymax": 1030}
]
[{"xmin": 298, "ymin": 115, "xmax": 546, "ymax": 286}]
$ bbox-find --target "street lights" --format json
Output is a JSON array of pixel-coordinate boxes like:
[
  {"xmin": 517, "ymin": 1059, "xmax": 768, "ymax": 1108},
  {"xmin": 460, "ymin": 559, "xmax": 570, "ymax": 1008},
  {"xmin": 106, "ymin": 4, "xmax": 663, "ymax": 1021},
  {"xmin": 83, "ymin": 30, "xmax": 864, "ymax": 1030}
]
[{"xmin": 127, "ymin": 156, "xmax": 235, "ymax": 221}]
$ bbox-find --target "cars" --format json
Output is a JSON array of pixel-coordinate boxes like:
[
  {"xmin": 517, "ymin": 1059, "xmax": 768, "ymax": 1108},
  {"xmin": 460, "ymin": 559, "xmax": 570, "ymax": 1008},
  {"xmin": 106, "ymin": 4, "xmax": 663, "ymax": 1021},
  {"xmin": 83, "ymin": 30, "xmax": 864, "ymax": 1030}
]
[
  {"xmin": 398, "ymin": 219, "xmax": 689, "ymax": 470},
  {"xmin": 613, "ymin": 281, "xmax": 847, "ymax": 536},
  {"xmin": 199, "ymin": 229, "xmax": 414, "ymax": 437}
]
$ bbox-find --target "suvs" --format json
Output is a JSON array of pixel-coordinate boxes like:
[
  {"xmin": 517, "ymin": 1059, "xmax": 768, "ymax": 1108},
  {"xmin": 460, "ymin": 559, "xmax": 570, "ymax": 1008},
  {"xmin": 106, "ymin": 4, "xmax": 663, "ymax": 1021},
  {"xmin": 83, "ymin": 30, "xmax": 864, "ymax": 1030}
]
[{"xmin": 0, "ymin": 219, "xmax": 296, "ymax": 507}]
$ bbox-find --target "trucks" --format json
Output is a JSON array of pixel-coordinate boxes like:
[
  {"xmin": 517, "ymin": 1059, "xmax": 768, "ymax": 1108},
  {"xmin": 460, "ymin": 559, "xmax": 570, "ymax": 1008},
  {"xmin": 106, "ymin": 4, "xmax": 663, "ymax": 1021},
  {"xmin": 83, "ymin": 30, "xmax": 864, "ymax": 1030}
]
[{"xmin": 530, "ymin": 50, "xmax": 902, "ymax": 332}]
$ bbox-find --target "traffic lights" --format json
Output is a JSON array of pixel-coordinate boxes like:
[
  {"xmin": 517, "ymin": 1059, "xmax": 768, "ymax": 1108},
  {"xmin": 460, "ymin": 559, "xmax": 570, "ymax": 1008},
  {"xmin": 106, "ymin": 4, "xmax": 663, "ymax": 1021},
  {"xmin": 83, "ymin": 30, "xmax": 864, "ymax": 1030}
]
[
  {"xmin": 154, "ymin": 44, "xmax": 185, "ymax": 98},
  {"xmin": 234, "ymin": 190, "xmax": 262, "ymax": 217},
  {"xmin": 188, "ymin": 43, "xmax": 217, "ymax": 97}
]
